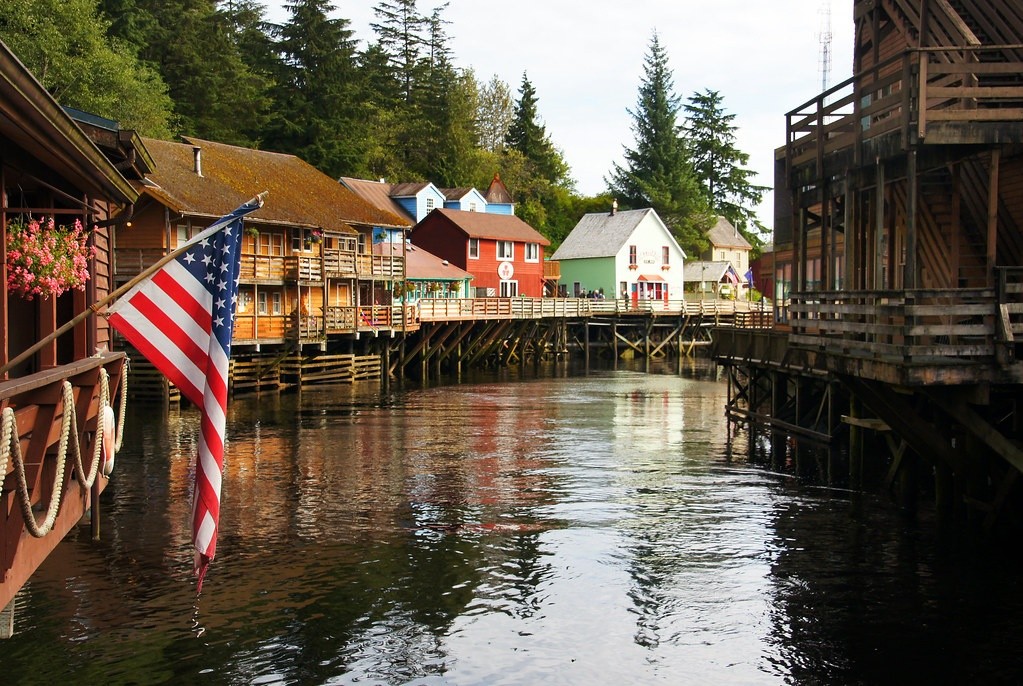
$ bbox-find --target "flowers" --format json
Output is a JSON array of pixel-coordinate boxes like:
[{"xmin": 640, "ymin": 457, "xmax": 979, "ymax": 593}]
[
  {"xmin": 308, "ymin": 228, "xmax": 322, "ymax": 245},
  {"xmin": 6, "ymin": 214, "xmax": 100, "ymax": 302}
]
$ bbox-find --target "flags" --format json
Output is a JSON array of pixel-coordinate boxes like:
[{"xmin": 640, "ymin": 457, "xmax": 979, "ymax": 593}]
[
  {"xmin": 100, "ymin": 199, "xmax": 263, "ymax": 594},
  {"xmin": 725, "ymin": 268, "xmax": 739, "ymax": 286},
  {"xmin": 744, "ymin": 268, "xmax": 753, "ymax": 288}
]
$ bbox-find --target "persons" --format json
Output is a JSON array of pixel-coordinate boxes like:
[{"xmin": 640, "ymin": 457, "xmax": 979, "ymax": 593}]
[
  {"xmin": 625, "ymin": 292, "xmax": 629, "ymax": 311},
  {"xmin": 580, "ymin": 289, "xmax": 605, "ymax": 312}
]
